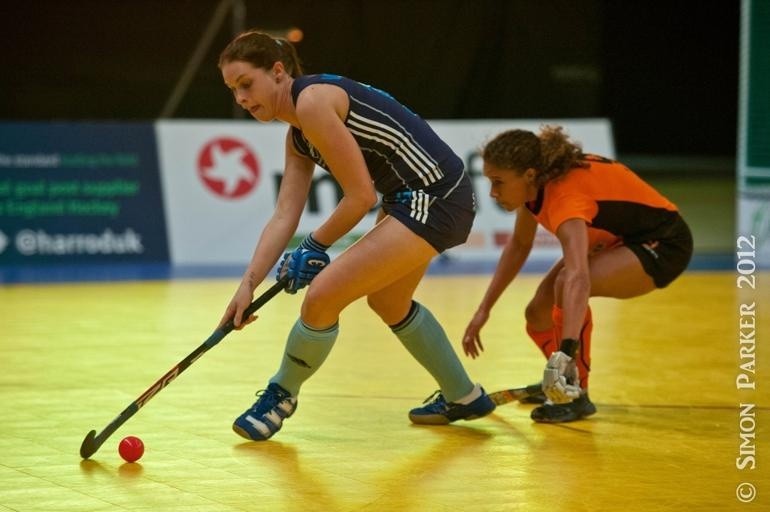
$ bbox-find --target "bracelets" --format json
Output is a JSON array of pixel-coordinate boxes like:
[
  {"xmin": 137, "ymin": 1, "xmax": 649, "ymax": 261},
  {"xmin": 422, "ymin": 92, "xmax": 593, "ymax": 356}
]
[{"xmin": 559, "ymin": 339, "xmax": 579, "ymax": 359}]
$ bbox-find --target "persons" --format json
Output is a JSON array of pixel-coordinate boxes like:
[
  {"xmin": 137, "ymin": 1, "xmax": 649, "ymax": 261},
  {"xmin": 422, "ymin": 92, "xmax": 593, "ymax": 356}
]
[
  {"xmin": 216, "ymin": 31, "xmax": 495, "ymax": 442},
  {"xmin": 461, "ymin": 123, "xmax": 695, "ymax": 422}
]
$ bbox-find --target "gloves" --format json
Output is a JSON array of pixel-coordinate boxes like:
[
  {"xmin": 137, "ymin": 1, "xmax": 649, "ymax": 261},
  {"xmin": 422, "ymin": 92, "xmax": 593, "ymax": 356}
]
[
  {"xmin": 276, "ymin": 231, "xmax": 330, "ymax": 295},
  {"xmin": 541, "ymin": 351, "xmax": 584, "ymax": 404}
]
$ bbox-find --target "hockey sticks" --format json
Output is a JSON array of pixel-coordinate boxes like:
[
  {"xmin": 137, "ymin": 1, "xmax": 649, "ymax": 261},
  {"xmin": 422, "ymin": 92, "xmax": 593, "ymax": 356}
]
[{"xmin": 79, "ymin": 274, "xmax": 289, "ymax": 459}]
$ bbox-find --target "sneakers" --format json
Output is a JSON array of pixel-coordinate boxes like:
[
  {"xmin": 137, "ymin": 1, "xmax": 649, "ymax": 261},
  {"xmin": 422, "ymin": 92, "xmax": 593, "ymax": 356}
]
[
  {"xmin": 234, "ymin": 381, "xmax": 298, "ymax": 441},
  {"xmin": 408, "ymin": 384, "xmax": 494, "ymax": 424},
  {"xmin": 530, "ymin": 394, "xmax": 596, "ymax": 423}
]
